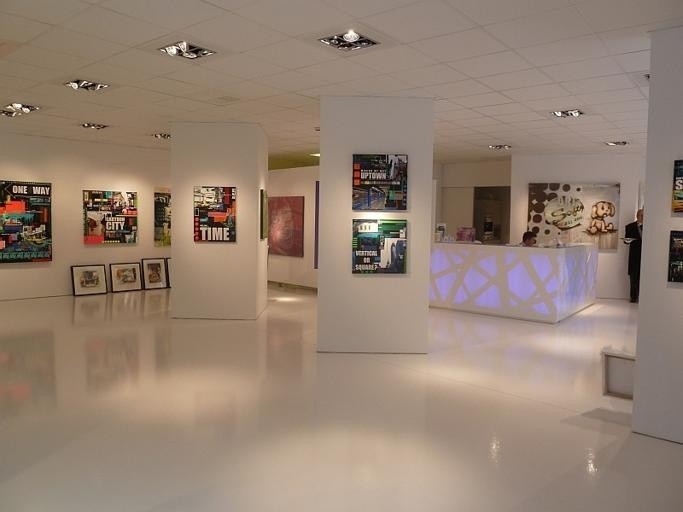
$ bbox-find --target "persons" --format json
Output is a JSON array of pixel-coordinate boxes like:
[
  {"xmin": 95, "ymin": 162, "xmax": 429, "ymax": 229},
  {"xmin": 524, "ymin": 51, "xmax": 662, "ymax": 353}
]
[
  {"xmin": 623, "ymin": 209, "xmax": 644, "ymax": 302},
  {"xmin": 156, "ymin": 197, "xmax": 172, "ymax": 221},
  {"xmin": 518, "ymin": 231, "xmax": 536, "ymax": 247}
]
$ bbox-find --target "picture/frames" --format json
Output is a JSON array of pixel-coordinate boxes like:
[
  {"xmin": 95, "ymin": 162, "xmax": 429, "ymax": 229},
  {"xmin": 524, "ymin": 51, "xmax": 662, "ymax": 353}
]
[{"xmin": 69, "ymin": 256, "xmax": 172, "ymax": 297}]
